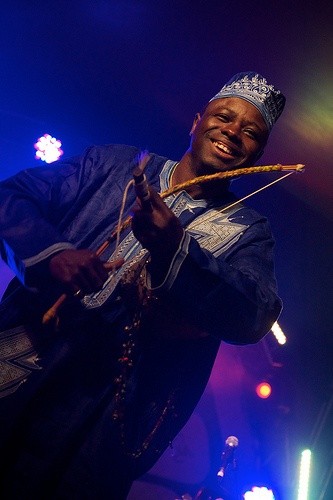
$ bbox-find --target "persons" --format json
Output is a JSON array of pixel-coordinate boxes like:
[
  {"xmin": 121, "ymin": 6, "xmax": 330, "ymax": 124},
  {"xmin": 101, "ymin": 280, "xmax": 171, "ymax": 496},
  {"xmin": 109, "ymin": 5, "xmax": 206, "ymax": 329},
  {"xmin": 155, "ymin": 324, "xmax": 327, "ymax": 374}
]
[{"xmin": 0, "ymin": 69, "xmax": 285, "ymax": 498}]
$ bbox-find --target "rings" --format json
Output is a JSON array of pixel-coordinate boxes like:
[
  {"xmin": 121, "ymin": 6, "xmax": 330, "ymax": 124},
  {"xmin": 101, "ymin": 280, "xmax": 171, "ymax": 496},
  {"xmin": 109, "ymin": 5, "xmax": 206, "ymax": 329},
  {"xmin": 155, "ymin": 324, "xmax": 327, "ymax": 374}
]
[{"xmin": 73, "ymin": 289, "xmax": 81, "ymax": 297}]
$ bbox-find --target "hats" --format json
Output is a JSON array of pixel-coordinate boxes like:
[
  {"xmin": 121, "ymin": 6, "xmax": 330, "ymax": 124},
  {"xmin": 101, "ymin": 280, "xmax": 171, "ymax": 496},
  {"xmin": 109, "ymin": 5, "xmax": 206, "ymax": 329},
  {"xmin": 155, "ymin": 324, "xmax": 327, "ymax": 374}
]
[{"xmin": 210, "ymin": 72, "xmax": 285, "ymax": 130}]
